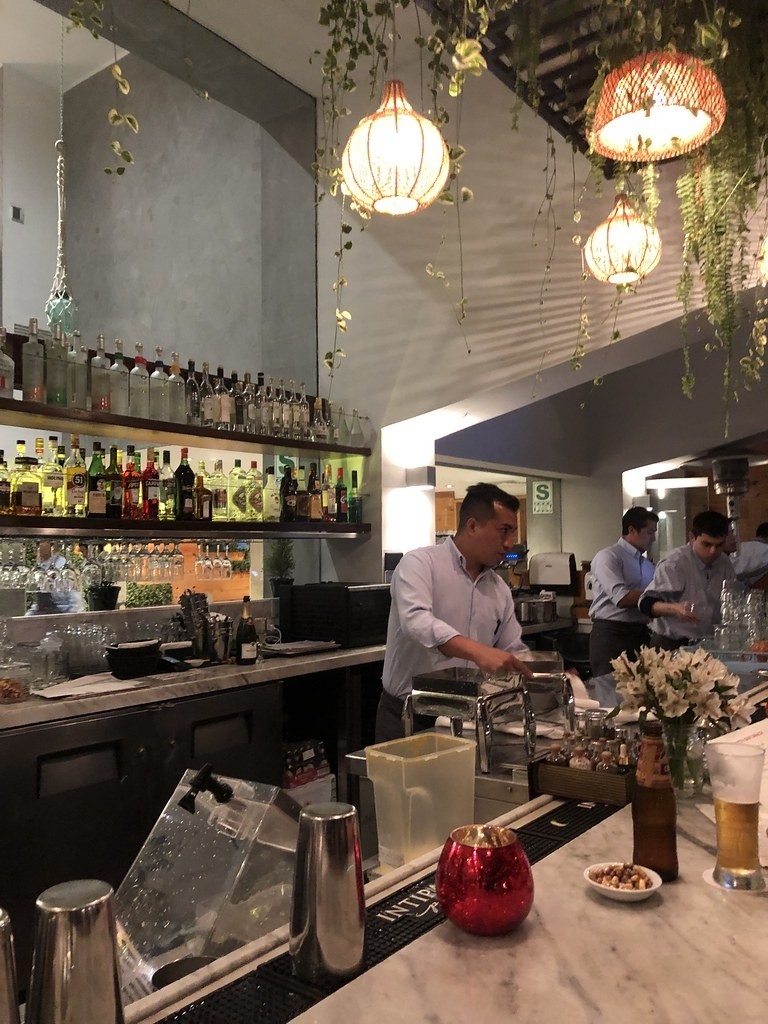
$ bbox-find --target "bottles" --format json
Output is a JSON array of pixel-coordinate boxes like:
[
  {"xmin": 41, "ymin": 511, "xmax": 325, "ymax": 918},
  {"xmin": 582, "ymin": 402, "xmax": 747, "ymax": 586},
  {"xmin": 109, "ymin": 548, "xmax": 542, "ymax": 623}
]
[
  {"xmin": 281, "ymin": 463, "xmax": 363, "ymax": 522},
  {"xmin": 44, "ymin": 323, "xmax": 87, "ymax": 410},
  {"xmin": 546, "ymin": 709, "xmax": 639, "ymax": 776},
  {"xmin": 631, "ymin": 723, "xmax": 679, "ymax": 882},
  {"xmin": 0, "ymin": 433, "xmax": 280, "ymax": 522},
  {"xmin": 236, "ymin": 596, "xmax": 258, "ymax": 665},
  {"xmin": 22, "ymin": 318, "xmax": 44, "ymax": 402},
  {"xmin": 326, "ymin": 401, "xmax": 338, "ymax": 444},
  {"xmin": 91, "ymin": 334, "xmax": 185, "ymax": 424},
  {"xmin": 337, "ymin": 407, "xmax": 350, "ymax": 446},
  {"xmin": 311, "ymin": 398, "xmax": 328, "ymax": 444},
  {"xmin": 185, "ymin": 359, "xmax": 310, "ymax": 440},
  {"xmin": 350, "ymin": 409, "xmax": 365, "ymax": 447},
  {"xmin": 29, "ymin": 633, "xmax": 70, "ymax": 684},
  {"xmin": 0, "ymin": 327, "xmax": 15, "ymax": 398}
]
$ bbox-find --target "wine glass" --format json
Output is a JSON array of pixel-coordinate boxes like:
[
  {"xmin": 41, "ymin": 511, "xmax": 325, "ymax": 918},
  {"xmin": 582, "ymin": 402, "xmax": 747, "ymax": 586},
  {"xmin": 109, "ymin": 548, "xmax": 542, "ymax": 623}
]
[
  {"xmin": 0, "ymin": 616, "xmax": 15, "ymax": 666},
  {"xmin": 0, "ymin": 538, "xmax": 184, "ymax": 591},
  {"xmin": 195, "ymin": 539, "xmax": 232, "ymax": 581}
]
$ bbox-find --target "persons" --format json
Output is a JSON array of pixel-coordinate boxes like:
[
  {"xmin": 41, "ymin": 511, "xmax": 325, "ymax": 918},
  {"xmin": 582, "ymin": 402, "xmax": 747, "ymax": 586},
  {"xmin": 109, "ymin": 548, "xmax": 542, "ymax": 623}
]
[
  {"xmin": 588, "ymin": 505, "xmax": 768, "ymax": 676},
  {"xmin": 372, "ymin": 482, "xmax": 536, "ymax": 745}
]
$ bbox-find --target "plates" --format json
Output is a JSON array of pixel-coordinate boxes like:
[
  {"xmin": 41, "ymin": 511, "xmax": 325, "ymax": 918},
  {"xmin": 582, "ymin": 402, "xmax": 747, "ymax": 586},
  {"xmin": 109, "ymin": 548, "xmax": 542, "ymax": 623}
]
[{"xmin": 585, "ymin": 861, "xmax": 662, "ymax": 902}]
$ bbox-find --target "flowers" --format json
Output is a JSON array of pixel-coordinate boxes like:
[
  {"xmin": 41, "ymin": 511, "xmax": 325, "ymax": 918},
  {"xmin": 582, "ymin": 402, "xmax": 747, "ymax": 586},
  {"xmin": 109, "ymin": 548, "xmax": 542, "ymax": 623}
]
[{"xmin": 604, "ymin": 645, "xmax": 756, "ymax": 731}]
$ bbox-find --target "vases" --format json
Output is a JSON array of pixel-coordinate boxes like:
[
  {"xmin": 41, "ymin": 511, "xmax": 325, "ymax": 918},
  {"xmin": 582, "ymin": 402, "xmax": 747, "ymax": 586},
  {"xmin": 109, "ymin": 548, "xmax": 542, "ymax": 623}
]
[{"xmin": 659, "ymin": 720, "xmax": 704, "ymax": 799}]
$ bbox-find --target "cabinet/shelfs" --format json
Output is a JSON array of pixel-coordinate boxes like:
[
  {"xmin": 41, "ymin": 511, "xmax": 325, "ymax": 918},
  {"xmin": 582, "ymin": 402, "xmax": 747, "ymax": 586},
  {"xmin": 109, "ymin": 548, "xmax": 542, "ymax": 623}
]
[{"xmin": 0, "ymin": 396, "xmax": 372, "ymax": 540}]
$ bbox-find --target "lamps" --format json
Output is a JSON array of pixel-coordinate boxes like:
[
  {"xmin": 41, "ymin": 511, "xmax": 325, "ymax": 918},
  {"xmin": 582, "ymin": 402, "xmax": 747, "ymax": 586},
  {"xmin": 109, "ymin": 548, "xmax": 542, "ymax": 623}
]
[
  {"xmin": 586, "ymin": 0, "xmax": 726, "ymax": 162},
  {"xmin": 584, "ymin": 191, "xmax": 662, "ymax": 284},
  {"xmin": 342, "ymin": 0, "xmax": 451, "ymax": 216}
]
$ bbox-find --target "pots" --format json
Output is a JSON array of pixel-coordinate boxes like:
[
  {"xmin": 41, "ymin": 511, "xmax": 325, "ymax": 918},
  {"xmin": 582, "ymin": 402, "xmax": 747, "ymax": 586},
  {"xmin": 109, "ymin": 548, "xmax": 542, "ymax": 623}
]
[
  {"xmin": 104, "ymin": 638, "xmax": 162, "ymax": 680},
  {"xmin": 517, "ymin": 599, "xmax": 557, "ymax": 623}
]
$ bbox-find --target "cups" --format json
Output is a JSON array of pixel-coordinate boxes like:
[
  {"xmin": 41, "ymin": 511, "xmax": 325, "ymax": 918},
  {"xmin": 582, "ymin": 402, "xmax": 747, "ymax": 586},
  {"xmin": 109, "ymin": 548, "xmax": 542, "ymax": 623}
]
[
  {"xmin": 0, "ymin": 908, "xmax": 21, "ymax": 1024},
  {"xmin": 714, "ymin": 579, "xmax": 768, "ymax": 660},
  {"xmin": 706, "ymin": 743, "xmax": 765, "ymax": 890},
  {"xmin": 53, "ymin": 622, "xmax": 175, "ymax": 666},
  {"xmin": 436, "ymin": 825, "xmax": 535, "ymax": 936},
  {"xmin": 24, "ymin": 880, "xmax": 124, "ymax": 1024},
  {"xmin": 289, "ymin": 803, "xmax": 365, "ymax": 977}
]
